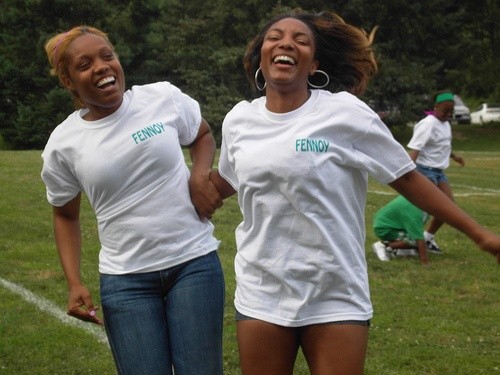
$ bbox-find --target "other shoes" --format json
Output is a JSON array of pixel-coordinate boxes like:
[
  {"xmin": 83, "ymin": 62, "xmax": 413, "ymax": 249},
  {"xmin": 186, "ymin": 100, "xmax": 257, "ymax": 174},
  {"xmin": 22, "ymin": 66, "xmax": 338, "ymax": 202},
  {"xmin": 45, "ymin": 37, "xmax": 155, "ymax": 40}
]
[
  {"xmin": 396, "ymin": 249, "xmax": 417, "ymax": 256},
  {"xmin": 373, "ymin": 240, "xmax": 390, "ymax": 262},
  {"xmin": 424, "ymin": 239, "xmax": 444, "ymax": 254}
]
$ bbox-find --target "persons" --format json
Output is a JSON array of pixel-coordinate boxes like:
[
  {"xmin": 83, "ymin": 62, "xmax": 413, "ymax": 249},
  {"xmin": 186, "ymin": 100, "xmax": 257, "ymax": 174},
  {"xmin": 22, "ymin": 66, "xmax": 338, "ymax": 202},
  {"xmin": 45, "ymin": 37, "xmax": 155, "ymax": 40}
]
[
  {"xmin": 209, "ymin": 8, "xmax": 500, "ymax": 375},
  {"xmin": 39, "ymin": 26, "xmax": 225, "ymax": 375},
  {"xmin": 372, "ymin": 90, "xmax": 465, "ymax": 265}
]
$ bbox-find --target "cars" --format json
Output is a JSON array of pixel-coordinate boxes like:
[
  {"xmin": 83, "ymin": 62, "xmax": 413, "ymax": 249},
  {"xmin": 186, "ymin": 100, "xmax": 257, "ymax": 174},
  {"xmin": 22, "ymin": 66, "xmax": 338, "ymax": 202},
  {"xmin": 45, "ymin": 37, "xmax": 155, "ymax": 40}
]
[
  {"xmin": 471, "ymin": 102, "xmax": 500, "ymax": 125},
  {"xmin": 452, "ymin": 94, "xmax": 472, "ymax": 124}
]
